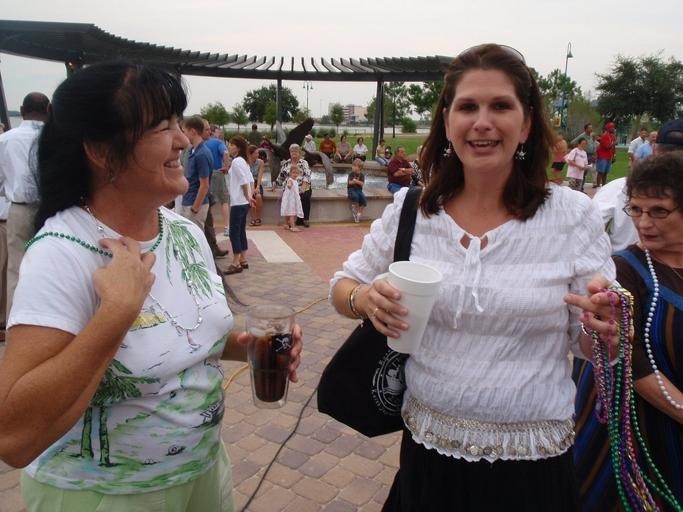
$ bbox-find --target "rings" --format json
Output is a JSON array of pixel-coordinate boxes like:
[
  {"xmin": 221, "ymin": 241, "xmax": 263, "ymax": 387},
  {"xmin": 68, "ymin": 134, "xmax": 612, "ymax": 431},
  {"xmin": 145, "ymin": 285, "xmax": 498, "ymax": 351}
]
[{"xmin": 372, "ymin": 307, "xmax": 379, "ymax": 316}]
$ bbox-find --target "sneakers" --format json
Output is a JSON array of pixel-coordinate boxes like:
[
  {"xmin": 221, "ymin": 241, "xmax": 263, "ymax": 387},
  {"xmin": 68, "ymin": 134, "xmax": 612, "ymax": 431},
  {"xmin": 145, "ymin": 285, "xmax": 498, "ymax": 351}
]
[
  {"xmin": 255, "ymin": 218, "xmax": 263, "ymax": 226},
  {"xmin": 296, "ymin": 220, "xmax": 303, "ymax": 225},
  {"xmin": 224, "ymin": 230, "xmax": 230, "ymax": 235},
  {"xmin": 352, "ymin": 204, "xmax": 357, "ymax": 217},
  {"xmin": 355, "ymin": 214, "xmax": 360, "ymax": 223},
  {"xmin": 249, "ymin": 220, "xmax": 255, "ymax": 226},
  {"xmin": 303, "ymin": 220, "xmax": 310, "ymax": 227},
  {"xmin": 215, "ymin": 245, "xmax": 229, "ymax": 256}
]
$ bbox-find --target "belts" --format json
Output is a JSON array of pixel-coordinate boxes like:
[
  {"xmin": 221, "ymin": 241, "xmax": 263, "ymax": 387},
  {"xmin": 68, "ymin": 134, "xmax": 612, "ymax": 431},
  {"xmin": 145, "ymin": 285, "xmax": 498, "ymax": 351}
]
[
  {"xmin": 11, "ymin": 201, "xmax": 33, "ymax": 206},
  {"xmin": 0, "ymin": 219, "xmax": 9, "ymax": 223}
]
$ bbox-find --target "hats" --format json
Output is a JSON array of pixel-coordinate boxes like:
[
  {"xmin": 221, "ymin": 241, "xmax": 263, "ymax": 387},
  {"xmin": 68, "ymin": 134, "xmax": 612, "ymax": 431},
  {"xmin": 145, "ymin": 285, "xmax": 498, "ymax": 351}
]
[
  {"xmin": 605, "ymin": 122, "xmax": 615, "ymax": 129},
  {"xmin": 656, "ymin": 119, "xmax": 683, "ymax": 145},
  {"xmin": 305, "ymin": 135, "xmax": 312, "ymax": 140}
]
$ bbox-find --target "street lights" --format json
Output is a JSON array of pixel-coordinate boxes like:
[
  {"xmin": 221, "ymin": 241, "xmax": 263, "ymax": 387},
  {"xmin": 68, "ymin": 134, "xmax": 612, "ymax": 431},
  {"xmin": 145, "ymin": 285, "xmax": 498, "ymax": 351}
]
[
  {"xmin": 561, "ymin": 42, "xmax": 575, "ymax": 120},
  {"xmin": 303, "ymin": 81, "xmax": 313, "ymax": 117}
]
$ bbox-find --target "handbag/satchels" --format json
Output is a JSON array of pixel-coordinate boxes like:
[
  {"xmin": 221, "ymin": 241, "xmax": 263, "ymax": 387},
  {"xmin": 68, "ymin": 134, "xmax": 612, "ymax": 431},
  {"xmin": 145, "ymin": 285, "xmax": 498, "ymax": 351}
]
[
  {"xmin": 317, "ymin": 186, "xmax": 424, "ymax": 437},
  {"xmin": 564, "ymin": 150, "xmax": 576, "ymax": 166}
]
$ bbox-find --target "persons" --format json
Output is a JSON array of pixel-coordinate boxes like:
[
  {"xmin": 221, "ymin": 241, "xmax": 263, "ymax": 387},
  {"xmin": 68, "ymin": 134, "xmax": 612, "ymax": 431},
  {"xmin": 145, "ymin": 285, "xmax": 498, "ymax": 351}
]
[
  {"xmin": 563, "ymin": 137, "xmax": 593, "ymax": 192},
  {"xmin": 203, "ymin": 126, "xmax": 230, "ymax": 236},
  {"xmin": 551, "ymin": 134, "xmax": 568, "ymax": 184},
  {"xmin": 280, "ymin": 166, "xmax": 304, "ymax": 232},
  {"xmin": 597, "ymin": 122, "xmax": 618, "ymax": 187},
  {"xmin": 627, "ymin": 128, "xmax": 653, "ymax": 170},
  {"xmin": 569, "ymin": 150, "xmax": 683, "ymax": 512},
  {"xmin": 570, "ymin": 123, "xmax": 593, "ymax": 194},
  {"xmin": 200, "ymin": 119, "xmax": 228, "ymax": 260},
  {"xmin": 276, "ymin": 143, "xmax": 312, "ymax": 227},
  {"xmin": 375, "ymin": 140, "xmax": 390, "ymax": 169},
  {"xmin": 347, "ymin": 159, "xmax": 368, "ymax": 223},
  {"xmin": 1, "ymin": 123, "xmax": 11, "ymax": 341},
  {"xmin": 301, "ymin": 134, "xmax": 316, "ymax": 167},
  {"xmin": 246, "ymin": 144, "xmax": 265, "ymax": 225},
  {"xmin": 331, "ymin": 135, "xmax": 352, "ymax": 164},
  {"xmin": 592, "ymin": 120, "xmax": 683, "ymax": 253},
  {"xmin": 315, "ymin": 133, "xmax": 336, "ymax": 161},
  {"xmin": 590, "ymin": 132, "xmax": 601, "ymax": 188},
  {"xmin": 385, "ymin": 146, "xmax": 392, "ymax": 159},
  {"xmin": 353, "ymin": 137, "xmax": 368, "ymax": 162},
  {"xmin": 180, "ymin": 117, "xmax": 214, "ymax": 235},
  {"xmin": 328, "ymin": 45, "xmax": 621, "ymax": 512},
  {"xmin": 647, "ymin": 131, "xmax": 659, "ymax": 156},
  {"xmin": 0, "ymin": 93, "xmax": 51, "ymax": 326},
  {"xmin": 410, "ymin": 145, "xmax": 434, "ymax": 187},
  {"xmin": 223, "ymin": 135, "xmax": 257, "ymax": 274},
  {"xmin": 595, "ymin": 135, "xmax": 601, "ymax": 141},
  {"xmin": 1, "ymin": 60, "xmax": 302, "ymax": 512},
  {"xmin": 387, "ymin": 147, "xmax": 416, "ymax": 193},
  {"xmin": 247, "ymin": 124, "xmax": 262, "ymax": 148}
]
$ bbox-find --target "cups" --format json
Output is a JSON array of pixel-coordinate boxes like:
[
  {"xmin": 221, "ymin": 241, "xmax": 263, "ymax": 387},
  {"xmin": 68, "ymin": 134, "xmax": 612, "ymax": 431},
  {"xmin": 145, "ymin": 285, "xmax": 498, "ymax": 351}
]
[
  {"xmin": 388, "ymin": 262, "xmax": 442, "ymax": 354},
  {"xmin": 245, "ymin": 305, "xmax": 294, "ymax": 409}
]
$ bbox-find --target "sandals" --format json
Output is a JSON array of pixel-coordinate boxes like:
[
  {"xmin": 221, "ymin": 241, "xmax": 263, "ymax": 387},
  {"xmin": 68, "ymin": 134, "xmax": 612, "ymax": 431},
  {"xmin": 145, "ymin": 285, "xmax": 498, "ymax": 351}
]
[
  {"xmin": 290, "ymin": 228, "xmax": 299, "ymax": 232},
  {"xmin": 284, "ymin": 225, "xmax": 291, "ymax": 230},
  {"xmin": 240, "ymin": 261, "xmax": 248, "ymax": 268},
  {"xmin": 222, "ymin": 264, "xmax": 243, "ymax": 275}
]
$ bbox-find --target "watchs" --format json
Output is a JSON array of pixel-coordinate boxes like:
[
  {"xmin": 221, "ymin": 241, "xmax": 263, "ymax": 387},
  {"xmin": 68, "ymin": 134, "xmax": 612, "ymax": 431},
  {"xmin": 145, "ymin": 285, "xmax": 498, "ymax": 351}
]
[
  {"xmin": 403, "ymin": 168, "xmax": 406, "ymax": 172},
  {"xmin": 191, "ymin": 207, "xmax": 198, "ymax": 214}
]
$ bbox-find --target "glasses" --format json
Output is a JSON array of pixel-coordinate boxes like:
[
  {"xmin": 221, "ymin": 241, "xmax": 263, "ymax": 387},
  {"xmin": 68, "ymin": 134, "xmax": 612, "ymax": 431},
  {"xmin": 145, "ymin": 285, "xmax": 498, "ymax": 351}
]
[
  {"xmin": 456, "ymin": 45, "xmax": 525, "ymax": 65},
  {"xmin": 623, "ymin": 204, "xmax": 682, "ymax": 219}
]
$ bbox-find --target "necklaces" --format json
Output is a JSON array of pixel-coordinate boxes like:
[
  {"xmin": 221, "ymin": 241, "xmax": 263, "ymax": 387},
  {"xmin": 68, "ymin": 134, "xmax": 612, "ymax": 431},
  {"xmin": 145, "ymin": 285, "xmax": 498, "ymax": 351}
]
[
  {"xmin": 79, "ymin": 195, "xmax": 203, "ymax": 330},
  {"xmin": 583, "ymin": 284, "xmax": 683, "ymax": 512},
  {"xmin": 23, "ymin": 207, "xmax": 164, "ymax": 259},
  {"xmin": 650, "ymin": 250, "xmax": 683, "ymax": 280},
  {"xmin": 642, "ymin": 245, "xmax": 683, "ymax": 409}
]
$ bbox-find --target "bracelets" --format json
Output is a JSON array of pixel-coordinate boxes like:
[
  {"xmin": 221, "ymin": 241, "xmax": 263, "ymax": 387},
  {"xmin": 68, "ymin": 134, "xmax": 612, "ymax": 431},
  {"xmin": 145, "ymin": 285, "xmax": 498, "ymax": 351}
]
[
  {"xmin": 347, "ymin": 283, "xmax": 367, "ymax": 329},
  {"xmin": 257, "ymin": 185, "xmax": 260, "ymax": 189},
  {"xmin": 577, "ymin": 320, "xmax": 589, "ymax": 337}
]
